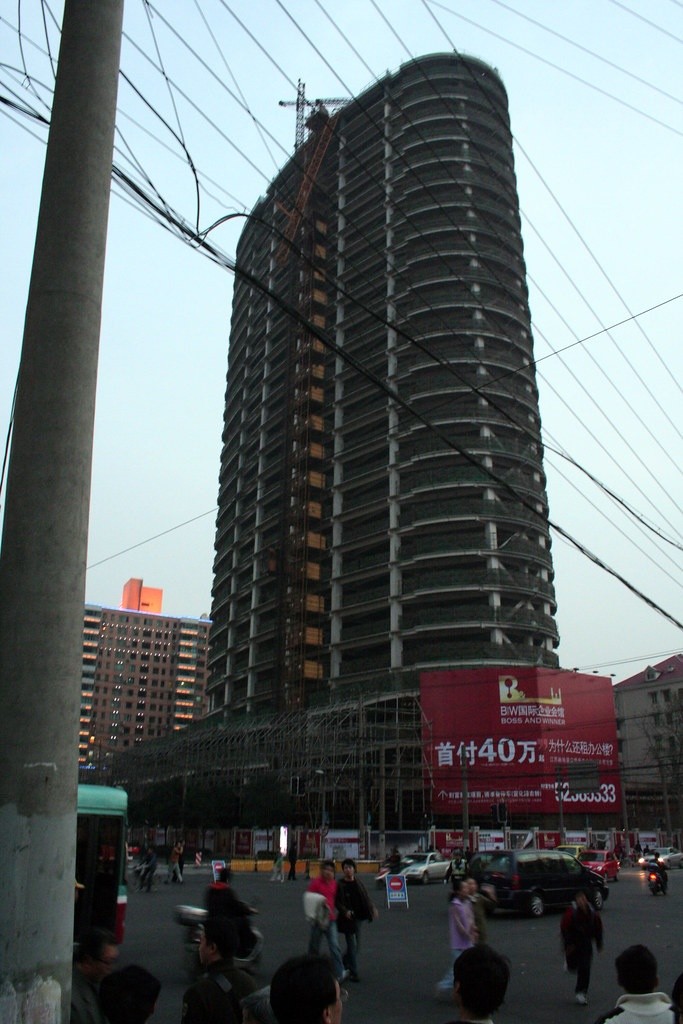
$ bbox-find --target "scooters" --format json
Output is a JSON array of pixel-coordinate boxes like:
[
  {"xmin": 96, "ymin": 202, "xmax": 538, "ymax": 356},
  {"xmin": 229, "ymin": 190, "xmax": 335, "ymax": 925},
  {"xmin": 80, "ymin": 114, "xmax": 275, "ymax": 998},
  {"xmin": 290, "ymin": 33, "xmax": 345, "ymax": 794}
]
[{"xmin": 374, "ymin": 862, "xmax": 392, "ymax": 889}]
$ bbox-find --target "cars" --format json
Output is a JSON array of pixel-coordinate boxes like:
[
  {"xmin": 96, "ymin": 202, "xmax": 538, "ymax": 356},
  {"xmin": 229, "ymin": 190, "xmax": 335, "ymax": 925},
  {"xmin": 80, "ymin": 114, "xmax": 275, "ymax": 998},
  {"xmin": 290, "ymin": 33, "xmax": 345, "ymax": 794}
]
[
  {"xmin": 638, "ymin": 846, "xmax": 683, "ymax": 870},
  {"xmin": 576, "ymin": 849, "xmax": 622, "ymax": 882},
  {"xmin": 399, "ymin": 852, "xmax": 456, "ymax": 884},
  {"xmin": 550, "ymin": 845, "xmax": 588, "ymax": 859}
]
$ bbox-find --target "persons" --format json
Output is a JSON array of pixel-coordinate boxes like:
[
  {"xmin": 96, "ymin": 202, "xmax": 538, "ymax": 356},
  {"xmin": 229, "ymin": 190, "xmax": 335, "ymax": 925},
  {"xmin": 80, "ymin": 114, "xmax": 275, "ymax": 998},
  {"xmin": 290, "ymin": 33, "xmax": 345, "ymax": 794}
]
[
  {"xmin": 334, "ymin": 859, "xmax": 378, "ymax": 982},
  {"xmin": 444, "ymin": 944, "xmax": 508, "ymax": 1024},
  {"xmin": 240, "ymin": 954, "xmax": 343, "ymax": 1024},
  {"xmin": 448, "ymin": 879, "xmax": 496, "ymax": 964},
  {"xmin": 304, "ymin": 860, "xmax": 349, "ymax": 982},
  {"xmin": 72, "ymin": 927, "xmax": 160, "ymax": 1024},
  {"xmin": 444, "ymin": 847, "xmax": 472, "ymax": 885},
  {"xmin": 206, "ymin": 868, "xmax": 258, "ymax": 919},
  {"xmin": 589, "ymin": 844, "xmax": 597, "ymax": 858},
  {"xmin": 650, "ymin": 853, "xmax": 667, "ymax": 884},
  {"xmin": 288, "ymin": 843, "xmax": 297, "ymax": 880},
  {"xmin": 593, "ymin": 944, "xmax": 683, "ymax": 1024},
  {"xmin": 560, "ymin": 891, "xmax": 603, "ymax": 1005},
  {"xmin": 428, "ymin": 846, "xmax": 434, "ymax": 851},
  {"xmin": 179, "ymin": 914, "xmax": 257, "ymax": 1024},
  {"xmin": 635, "ymin": 840, "xmax": 649, "ymax": 853},
  {"xmin": 164, "ymin": 840, "xmax": 186, "ymax": 884},
  {"xmin": 135, "ymin": 848, "xmax": 157, "ymax": 892},
  {"xmin": 220, "ymin": 865, "xmax": 232, "ymax": 883},
  {"xmin": 418, "ymin": 846, "xmax": 422, "ymax": 852},
  {"xmin": 386, "ymin": 850, "xmax": 400, "ymax": 863},
  {"xmin": 269, "ymin": 847, "xmax": 284, "ymax": 882}
]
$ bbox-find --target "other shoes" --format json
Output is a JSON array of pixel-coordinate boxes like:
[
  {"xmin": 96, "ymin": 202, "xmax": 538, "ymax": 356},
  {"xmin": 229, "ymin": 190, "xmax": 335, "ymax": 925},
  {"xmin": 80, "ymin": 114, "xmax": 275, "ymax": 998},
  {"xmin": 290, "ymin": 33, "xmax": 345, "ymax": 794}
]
[
  {"xmin": 564, "ymin": 961, "xmax": 569, "ymax": 973},
  {"xmin": 576, "ymin": 993, "xmax": 587, "ymax": 1005},
  {"xmin": 339, "ymin": 987, "xmax": 348, "ymax": 1002}
]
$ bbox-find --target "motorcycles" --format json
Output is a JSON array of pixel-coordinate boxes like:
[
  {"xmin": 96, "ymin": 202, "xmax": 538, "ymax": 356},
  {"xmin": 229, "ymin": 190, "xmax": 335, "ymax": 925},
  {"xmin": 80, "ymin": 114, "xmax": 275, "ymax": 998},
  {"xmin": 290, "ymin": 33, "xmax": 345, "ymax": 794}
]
[{"xmin": 643, "ymin": 858, "xmax": 670, "ymax": 895}]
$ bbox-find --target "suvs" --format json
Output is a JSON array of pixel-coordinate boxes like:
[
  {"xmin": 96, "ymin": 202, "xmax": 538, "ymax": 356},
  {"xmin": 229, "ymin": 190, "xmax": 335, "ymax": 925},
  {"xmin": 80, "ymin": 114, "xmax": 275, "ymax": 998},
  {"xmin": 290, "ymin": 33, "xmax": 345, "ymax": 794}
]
[{"xmin": 465, "ymin": 849, "xmax": 609, "ymax": 916}]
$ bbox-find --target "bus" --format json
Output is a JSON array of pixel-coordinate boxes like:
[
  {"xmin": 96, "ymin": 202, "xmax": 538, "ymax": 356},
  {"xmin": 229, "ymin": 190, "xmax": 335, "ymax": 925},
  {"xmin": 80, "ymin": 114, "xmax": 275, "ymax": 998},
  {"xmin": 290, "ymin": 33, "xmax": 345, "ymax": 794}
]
[{"xmin": 72, "ymin": 784, "xmax": 130, "ymax": 964}]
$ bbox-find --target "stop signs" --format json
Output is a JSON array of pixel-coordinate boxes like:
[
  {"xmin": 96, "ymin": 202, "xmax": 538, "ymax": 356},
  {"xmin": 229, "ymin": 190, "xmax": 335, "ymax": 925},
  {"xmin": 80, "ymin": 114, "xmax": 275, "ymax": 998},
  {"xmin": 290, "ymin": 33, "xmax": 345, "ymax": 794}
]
[
  {"xmin": 390, "ymin": 877, "xmax": 404, "ymax": 891},
  {"xmin": 215, "ymin": 863, "xmax": 223, "ymax": 872}
]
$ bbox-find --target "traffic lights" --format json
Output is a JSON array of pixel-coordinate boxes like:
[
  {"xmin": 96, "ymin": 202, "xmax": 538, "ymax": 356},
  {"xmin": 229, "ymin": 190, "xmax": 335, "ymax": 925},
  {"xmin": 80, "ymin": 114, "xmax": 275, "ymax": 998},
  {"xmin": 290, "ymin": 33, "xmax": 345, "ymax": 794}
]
[
  {"xmin": 297, "ymin": 777, "xmax": 306, "ymax": 797},
  {"xmin": 498, "ymin": 803, "xmax": 507, "ymax": 823},
  {"xmin": 290, "ymin": 777, "xmax": 299, "ymax": 796},
  {"xmin": 490, "ymin": 804, "xmax": 498, "ymax": 822}
]
